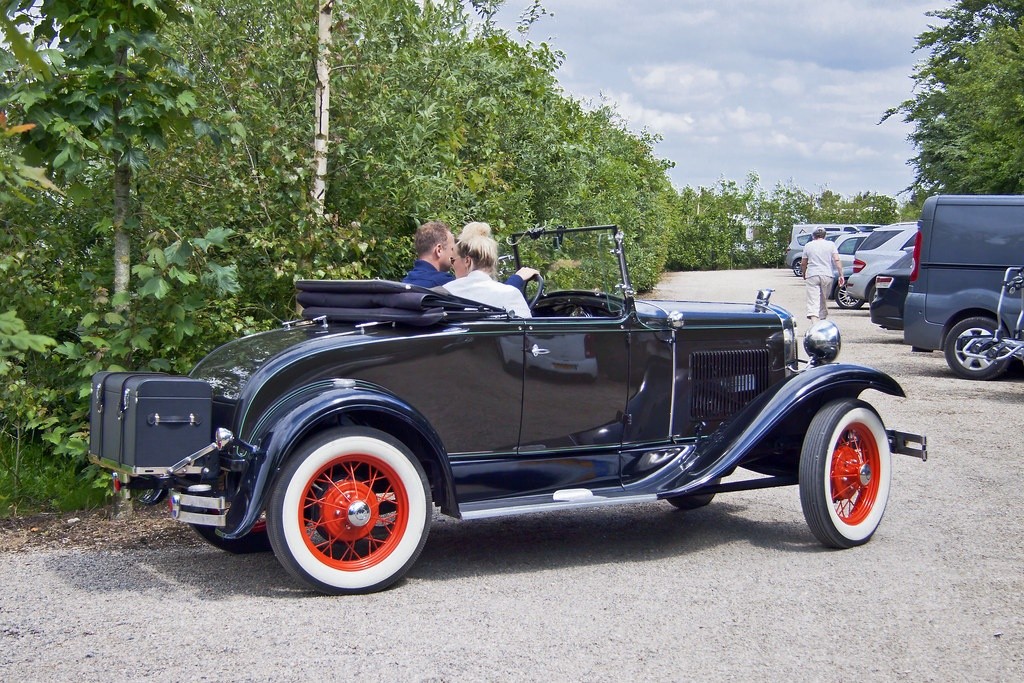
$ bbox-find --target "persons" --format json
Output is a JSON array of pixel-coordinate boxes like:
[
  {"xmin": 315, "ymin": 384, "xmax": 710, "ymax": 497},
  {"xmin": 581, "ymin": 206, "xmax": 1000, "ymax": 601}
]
[
  {"xmin": 402, "ymin": 222, "xmax": 539, "ymax": 294},
  {"xmin": 801, "ymin": 226, "xmax": 844, "ymax": 323},
  {"xmin": 443, "ymin": 222, "xmax": 533, "ymax": 318}
]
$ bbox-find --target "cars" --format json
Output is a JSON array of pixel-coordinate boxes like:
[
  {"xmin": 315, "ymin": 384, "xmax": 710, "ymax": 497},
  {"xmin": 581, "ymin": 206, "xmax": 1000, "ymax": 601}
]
[
  {"xmin": 787, "ymin": 217, "xmax": 919, "ymax": 309},
  {"xmin": 870, "ymin": 248, "xmax": 911, "ymax": 332},
  {"xmin": 86, "ymin": 223, "xmax": 928, "ymax": 598}
]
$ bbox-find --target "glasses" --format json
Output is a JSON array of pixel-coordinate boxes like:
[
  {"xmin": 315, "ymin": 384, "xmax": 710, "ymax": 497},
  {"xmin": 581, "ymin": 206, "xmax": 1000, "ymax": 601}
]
[{"xmin": 449, "ymin": 256, "xmax": 461, "ymax": 264}]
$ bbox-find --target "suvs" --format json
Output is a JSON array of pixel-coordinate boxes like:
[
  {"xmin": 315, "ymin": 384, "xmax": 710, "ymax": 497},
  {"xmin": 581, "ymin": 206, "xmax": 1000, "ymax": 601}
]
[{"xmin": 904, "ymin": 195, "xmax": 1023, "ymax": 382}]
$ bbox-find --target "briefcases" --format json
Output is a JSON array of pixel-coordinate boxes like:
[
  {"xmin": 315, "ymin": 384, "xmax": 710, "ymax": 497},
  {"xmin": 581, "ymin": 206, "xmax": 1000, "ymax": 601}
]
[{"xmin": 89, "ymin": 370, "xmax": 213, "ymax": 467}]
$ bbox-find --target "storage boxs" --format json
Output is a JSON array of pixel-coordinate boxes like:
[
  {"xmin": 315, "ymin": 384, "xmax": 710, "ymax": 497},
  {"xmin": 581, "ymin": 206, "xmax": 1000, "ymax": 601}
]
[{"xmin": 89, "ymin": 370, "xmax": 212, "ymax": 464}]
